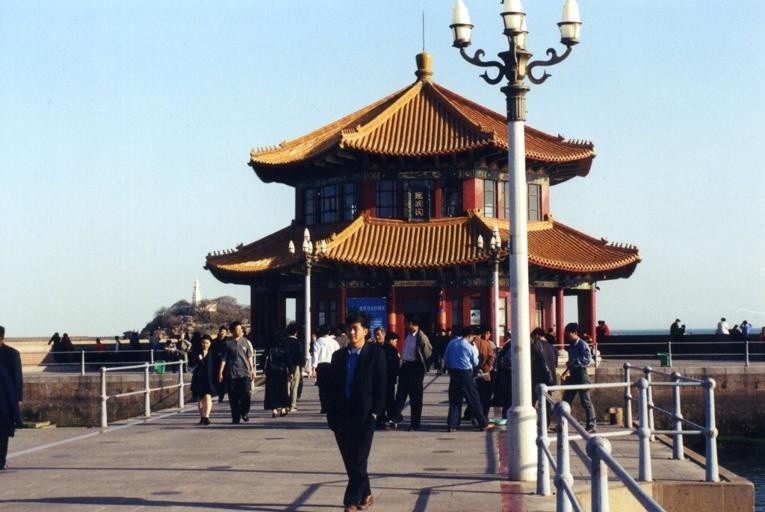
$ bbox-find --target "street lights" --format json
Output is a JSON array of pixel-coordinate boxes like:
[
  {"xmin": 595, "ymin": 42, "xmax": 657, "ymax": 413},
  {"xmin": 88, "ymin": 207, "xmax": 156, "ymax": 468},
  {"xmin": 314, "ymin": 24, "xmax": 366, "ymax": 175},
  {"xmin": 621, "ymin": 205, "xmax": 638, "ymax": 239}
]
[
  {"xmin": 448, "ymin": 1, "xmax": 586, "ymax": 485},
  {"xmin": 282, "ymin": 223, "xmax": 333, "ymax": 381},
  {"xmin": 474, "ymin": 219, "xmax": 510, "ymax": 348}
]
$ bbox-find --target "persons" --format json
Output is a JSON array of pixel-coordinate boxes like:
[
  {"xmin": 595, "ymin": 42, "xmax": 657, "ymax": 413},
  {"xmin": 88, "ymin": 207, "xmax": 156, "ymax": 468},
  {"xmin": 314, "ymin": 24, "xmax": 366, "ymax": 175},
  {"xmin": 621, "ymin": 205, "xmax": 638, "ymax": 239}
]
[
  {"xmin": 113, "ymin": 336, "xmax": 122, "ymax": 351},
  {"xmin": 0, "ymin": 326, "xmax": 22, "ymax": 470},
  {"xmin": 311, "ymin": 310, "xmax": 511, "ymax": 431},
  {"xmin": 326, "ymin": 315, "xmax": 387, "ymax": 512},
  {"xmin": 161, "ymin": 318, "xmax": 303, "ymax": 425},
  {"xmin": 94, "ymin": 339, "xmax": 105, "ymax": 360},
  {"xmin": 532, "ymin": 320, "xmax": 609, "ymax": 435},
  {"xmin": 670, "ymin": 316, "xmax": 686, "ymax": 340},
  {"xmin": 711, "ymin": 316, "xmax": 751, "ymax": 337},
  {"xmin": 63, "ymin": 333, "xmax": 73, "ymax": 361},
  {"xmin": 48, "ymin": 332, "xmax": 62, "ymax": 362}
]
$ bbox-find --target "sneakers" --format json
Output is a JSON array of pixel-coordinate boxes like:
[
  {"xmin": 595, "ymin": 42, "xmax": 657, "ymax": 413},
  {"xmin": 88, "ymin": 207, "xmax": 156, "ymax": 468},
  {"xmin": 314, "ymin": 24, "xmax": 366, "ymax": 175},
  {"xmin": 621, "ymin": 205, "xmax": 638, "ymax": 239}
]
[
  {"xmin": 199, "ymin": 416, "xmax": 211, "ymax": 425},
  {"xmin": 232, "ymin": 415, "xmax": 250, "ymax": 424},
  {"xmin": 479, "ymin": 423, "xmax": 496, "ymax": 431},
  {"xmin": 375, "ymin": 414, "xmax": 421, "ymax": 432},
  {"xmin": 550, "ymin": 424, "xmax": 597, "ymax": 434},
  {"xmin": 272, "ymin": 406, "xmax": 290, "ymax": 418},
  {"xmin": 357, "ymin": 493, "xmax": 374, "ymax": 510},
  {"xmin": 448, "ymin": 426, "xmax": 458, "ymax": 432},
  {"xmin": 344, "ymin": 505, "xmax": 358, "ymax": 512}
]
